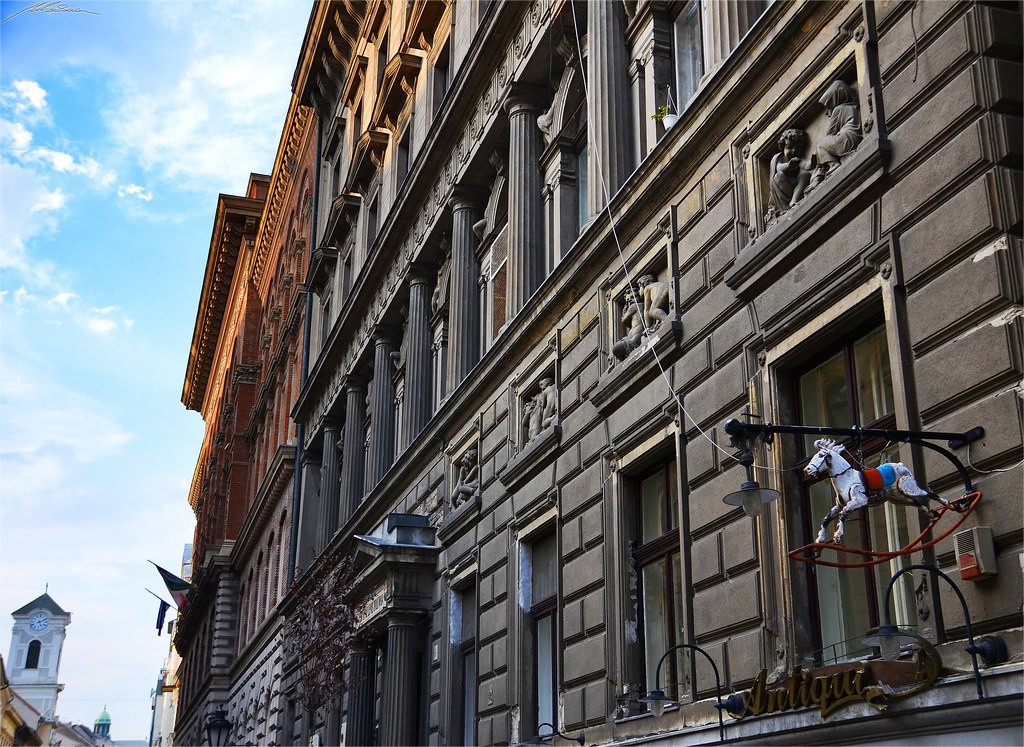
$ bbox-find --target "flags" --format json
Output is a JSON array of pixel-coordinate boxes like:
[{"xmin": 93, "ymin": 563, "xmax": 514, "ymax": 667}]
[
  {"xmin": 156, "ymin": 564, "xmax": 192, "ymax": 611},
  {"xmin": 156, "ymin": 600, "xmax": 170, "ymax": 636}
]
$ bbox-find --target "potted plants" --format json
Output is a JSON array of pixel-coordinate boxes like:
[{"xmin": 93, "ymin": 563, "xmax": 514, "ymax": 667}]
[{"xmin": 651, "ymin": 104, "xmax": 678, "ymax": 129}]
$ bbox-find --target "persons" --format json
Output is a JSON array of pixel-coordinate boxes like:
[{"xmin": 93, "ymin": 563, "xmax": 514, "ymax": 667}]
[
  {"xmin": 611, "ymin": 273, "xmax": 669, "ymax": 362},
  {"xmin": 452, "ymin": 449, "xmax": 478, "ymax": 508},
  {"xmin": 521, "ymin": 376, "xmax": 558, "ymax": 450},
  {"xmin": 765, "ymin": 80, "xmax": 863, "ymax": 216}
]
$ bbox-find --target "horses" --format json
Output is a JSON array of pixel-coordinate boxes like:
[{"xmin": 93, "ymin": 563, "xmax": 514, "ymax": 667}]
[{"xmin": 803, "ymin": 437, "xmax": 972, "ymax": 547}]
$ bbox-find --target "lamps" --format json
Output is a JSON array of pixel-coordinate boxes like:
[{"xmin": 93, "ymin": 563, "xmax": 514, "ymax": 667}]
[
  {"xmin": 722, "ymin": 419, "xmax": 985, "ymax": 518},
  {"xmin": 524, "ymin": 723, "xmax": 585, "ymax": 747},
  {"xmin": 203, "ymin": 703, "xmax": 258, "ymax": 747},
  {"xmin": 637, "ymin": 644, "xmax": 754, "ymax": 744},
  {"xmin": 859, "ymin": 564, "xmax": 1008, "ymax": 703}
]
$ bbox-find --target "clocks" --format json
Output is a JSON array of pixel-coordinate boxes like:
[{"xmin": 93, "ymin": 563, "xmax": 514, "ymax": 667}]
[{"xmin": 30, "ymin": 613, "xmax": 48, "ymax": 630}]
